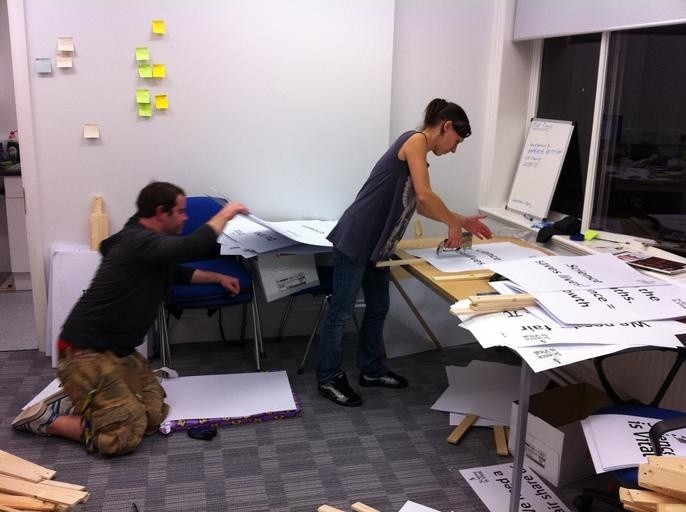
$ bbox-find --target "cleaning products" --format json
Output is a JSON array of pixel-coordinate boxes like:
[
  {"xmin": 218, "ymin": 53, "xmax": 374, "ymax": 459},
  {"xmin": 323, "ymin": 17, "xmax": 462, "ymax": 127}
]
[{"xmin": 6, "ymin": 130, "xmax": 20, "ymax": 165}]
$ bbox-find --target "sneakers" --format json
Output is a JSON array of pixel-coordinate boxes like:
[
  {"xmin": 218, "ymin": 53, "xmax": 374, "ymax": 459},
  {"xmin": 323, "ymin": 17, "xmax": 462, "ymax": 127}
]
[
  {"xmin": 9, "ymin": 389, "xmax": 79, "ymax": 438},
  {"xmin": 318, "ymin": 373, "xmax": 362, "ymax": 406},
  {"xmin": 358, "ymin": 369, "xmax": 410, "ymax": 388}
]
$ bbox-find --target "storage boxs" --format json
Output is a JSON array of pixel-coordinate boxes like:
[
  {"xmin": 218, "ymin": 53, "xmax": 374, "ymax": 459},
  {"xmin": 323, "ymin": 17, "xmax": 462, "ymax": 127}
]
[{"xmin": 510, "ymin": 383, "xmax": 624, "ymax": 487}]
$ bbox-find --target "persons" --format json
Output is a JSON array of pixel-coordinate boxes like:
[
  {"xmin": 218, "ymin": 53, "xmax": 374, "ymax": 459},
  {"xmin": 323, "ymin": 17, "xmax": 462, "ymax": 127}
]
[
  {"xmin": 316, "ymin": 99, "xmax": 492, "ymax": 407},
  {"xmin": 11, "ymin": 182, "xmax": 251, "ymax": 457}
]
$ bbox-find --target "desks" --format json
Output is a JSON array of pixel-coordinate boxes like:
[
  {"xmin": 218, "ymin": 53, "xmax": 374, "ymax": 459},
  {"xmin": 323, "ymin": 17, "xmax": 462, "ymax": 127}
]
[{"xmin": 383, "ymin": 234, "xmax": 686, "ymax": 512}]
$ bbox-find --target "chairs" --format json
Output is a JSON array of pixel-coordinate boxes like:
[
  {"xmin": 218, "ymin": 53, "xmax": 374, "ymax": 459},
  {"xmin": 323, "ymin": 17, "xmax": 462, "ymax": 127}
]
[
  {"xmin": 151, "ymin": 196, "xmax": 264, "ymax": 373},
  {"xmin": 280, "ymin": 212, "xmax": 387, "ymax": 375}
]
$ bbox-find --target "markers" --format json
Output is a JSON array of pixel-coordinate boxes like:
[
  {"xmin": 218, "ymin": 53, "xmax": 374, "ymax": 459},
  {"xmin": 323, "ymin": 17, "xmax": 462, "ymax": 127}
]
[{"xmin": 524, "ymin": 214, "xmax": 533, "ymax": 221}]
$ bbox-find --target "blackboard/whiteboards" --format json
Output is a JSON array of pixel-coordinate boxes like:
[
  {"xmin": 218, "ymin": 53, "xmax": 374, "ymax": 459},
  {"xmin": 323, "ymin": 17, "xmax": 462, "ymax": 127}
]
[{"xmin": 505, "ymin": 118, "xmax": 575, "ymax": 222}]
